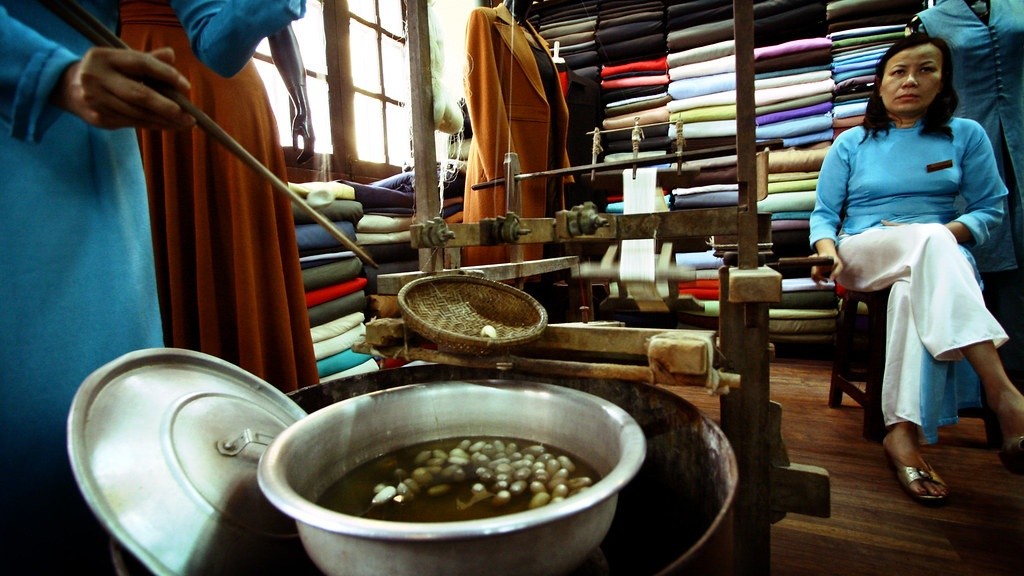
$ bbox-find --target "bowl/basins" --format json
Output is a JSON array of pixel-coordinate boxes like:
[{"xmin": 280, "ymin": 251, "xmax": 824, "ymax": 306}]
[{"xmin": 257, "ymin": 380, "xmax": 646, "ymax": 576}]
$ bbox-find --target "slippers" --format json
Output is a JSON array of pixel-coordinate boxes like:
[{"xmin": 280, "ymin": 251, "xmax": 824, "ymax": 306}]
[
  {"xmin": 999, "ymin": 435, "xmax": 1024, "ymax": 471},
  {"xmin": 888, "ymin": 454, "xmax": 945, "ymax": 504}
]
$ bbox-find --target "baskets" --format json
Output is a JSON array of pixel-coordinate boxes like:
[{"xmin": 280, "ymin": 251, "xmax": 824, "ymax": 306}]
[{"xmin": 397, "ymin": 274, "xmax": 548, "ymax": 357}]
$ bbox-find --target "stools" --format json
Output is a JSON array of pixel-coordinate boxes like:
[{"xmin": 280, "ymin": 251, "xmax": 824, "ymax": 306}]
[{"xmin": 829, "ymin": 287, "xmax": 1010, "ymax": 451}]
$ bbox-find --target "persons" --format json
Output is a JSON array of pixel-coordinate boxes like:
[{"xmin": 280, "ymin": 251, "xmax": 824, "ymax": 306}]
[
  {"xmin": 122, "ymin": 0, "xmax": 320, "ymax": 395},
  {"xmin": 0, "ymin": 0, "xmax": 189, "ymax": 576},
  {"xmin": 795, "ymin": 36, "xmax": 1024, "ymax": 503},
  {"xmin": 905, "ymin": 0, "xmax": 1024, "ymax": 397},
  {"xmin": 462, "ymin": 0, "xmax": 575, "ymax": 286}
]
